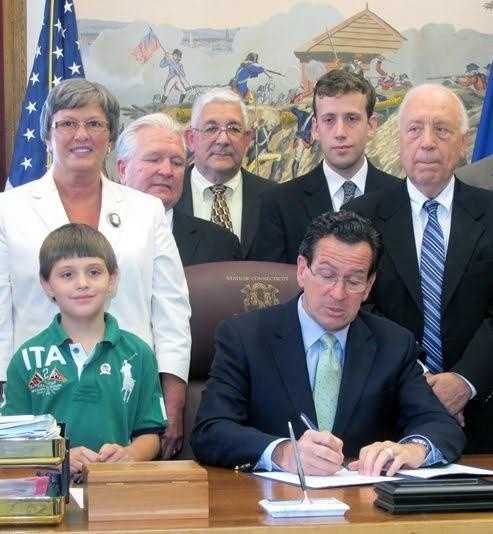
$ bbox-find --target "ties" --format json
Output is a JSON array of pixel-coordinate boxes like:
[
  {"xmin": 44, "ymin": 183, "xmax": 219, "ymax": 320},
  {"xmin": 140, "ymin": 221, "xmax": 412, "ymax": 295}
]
[
  {"xmin": 343, "ymin": 181, "xmax": 356, "ymax": 204},
  {"xmin": 420, "ymin": 200, "xmax": 446, "ymax": 374},
  {"xmin": 313, "ymin": 332, "xmax": 342, "ymax": 434},
  {"xmin": 209, "ymin": 184, "xmax": 233, "ymax": 233}
]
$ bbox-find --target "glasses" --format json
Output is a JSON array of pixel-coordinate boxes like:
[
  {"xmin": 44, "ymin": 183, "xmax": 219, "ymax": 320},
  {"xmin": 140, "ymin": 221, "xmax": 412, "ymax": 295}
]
[
  {"xmin": 193, "ymin": 123, "xmax": 248, "ymax": 141},
  {"xmin": 307, "ymin": 264, "xmax": 369, "ymax": 293},
  {"xmin": 49, "ymin": 119, "xmax": 110, "ymax": 132}
]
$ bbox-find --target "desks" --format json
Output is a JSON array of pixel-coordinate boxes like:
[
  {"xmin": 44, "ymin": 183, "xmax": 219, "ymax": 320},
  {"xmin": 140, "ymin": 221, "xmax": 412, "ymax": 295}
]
[{"xmin": 0, "ymin": 452, "xmax": 493, "ymax": 534}]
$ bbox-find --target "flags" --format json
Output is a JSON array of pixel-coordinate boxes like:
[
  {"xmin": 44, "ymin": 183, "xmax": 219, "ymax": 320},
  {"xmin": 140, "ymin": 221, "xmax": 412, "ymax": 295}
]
[
  {"xmin": 4, "ymin": 0, "xmax": 85, "ymax": 192},
  {"xmin": 130, "ymin": 31, "xmax": 160, "ymax": 65}
]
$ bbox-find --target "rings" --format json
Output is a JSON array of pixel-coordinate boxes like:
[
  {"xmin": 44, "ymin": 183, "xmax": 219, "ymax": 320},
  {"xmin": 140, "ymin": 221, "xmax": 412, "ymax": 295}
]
[{"xmin": 382, "ymin": 442, "xmax": 398, "ymax": 462}]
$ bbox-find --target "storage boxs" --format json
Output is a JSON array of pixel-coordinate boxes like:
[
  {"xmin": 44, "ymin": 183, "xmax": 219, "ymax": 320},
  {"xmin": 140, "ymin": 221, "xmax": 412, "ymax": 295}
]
[{"xmin": 81, "ymin": 461, "xmax": 208, "ymax": 529}]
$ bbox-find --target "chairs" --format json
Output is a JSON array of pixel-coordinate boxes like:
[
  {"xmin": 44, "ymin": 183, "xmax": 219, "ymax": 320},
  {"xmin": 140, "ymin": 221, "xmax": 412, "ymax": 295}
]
[{"xmin": 171, "ymin": 260, "xmax": 305, "ymax": 461}]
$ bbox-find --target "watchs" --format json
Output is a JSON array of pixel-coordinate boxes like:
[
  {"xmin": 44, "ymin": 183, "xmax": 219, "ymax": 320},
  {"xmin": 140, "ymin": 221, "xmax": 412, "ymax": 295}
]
[{"xmin": 401, "ymin": 435, "xmax": 432, "ymax": 456}]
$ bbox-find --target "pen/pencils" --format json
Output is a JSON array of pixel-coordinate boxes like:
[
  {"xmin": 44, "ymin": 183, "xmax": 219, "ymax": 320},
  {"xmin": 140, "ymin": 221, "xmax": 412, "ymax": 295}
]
[
  {"xmin": 288, "ymin": 422, "xmax": 311, "ymax": 504},
  {"xmin": 300, "ymin": 412, "xmax": 351, "ymax": 472}
]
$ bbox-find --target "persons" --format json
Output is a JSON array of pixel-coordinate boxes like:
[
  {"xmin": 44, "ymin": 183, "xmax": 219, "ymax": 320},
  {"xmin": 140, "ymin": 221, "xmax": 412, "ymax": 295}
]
[
  {"xmin": 159, "ymin": 48, "xmax": 187, "ymax": 104},
  {"xmin": 174, "ymin": 86, "xmax": 280, "ymax": 261},
  {"xmin": 0, "ymin": 76, "xmax": 193, "ymax": 462},
  {"xmin": 188, "ymin": 211, "xmax": 468, "ymax": 480},
  {"xmin": 229, "ymin": 53, "xmax": 265, "ymax": 99},
  {"xmin": 452, "ymin": 63, "xmax": 488, "ymax": 91},
  {"xmin": 391, "ymin": 73, "xmax": 413, "ymax": 91},
  {"xmin": 0, "ymin": 222, "xmax": 169, "ymax": 478},
  {"xmin": 250, "ymin": 68, "xmax": 402, "ymax": 263},
  {"xmin": 112, "ymin": 113, "xmax": 244, "ymax": 267},
  {"xmin": 349, "ymin": 57, "xmax": 364, "ymax": 77},
  {"xmin": 337, "ymin": 81, "xmax": 493, "ymax": 456}
]
